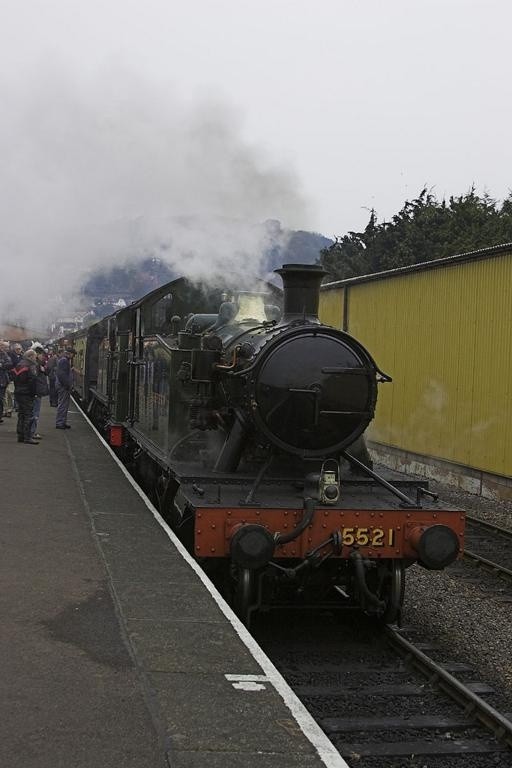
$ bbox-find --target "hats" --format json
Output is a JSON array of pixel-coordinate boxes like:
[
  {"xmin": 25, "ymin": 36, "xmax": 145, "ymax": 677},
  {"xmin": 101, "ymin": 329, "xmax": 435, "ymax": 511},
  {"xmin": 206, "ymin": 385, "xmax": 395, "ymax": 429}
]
[{"xmin": 66, "ymin": 347, "xmax": 77, "ymax": 354}]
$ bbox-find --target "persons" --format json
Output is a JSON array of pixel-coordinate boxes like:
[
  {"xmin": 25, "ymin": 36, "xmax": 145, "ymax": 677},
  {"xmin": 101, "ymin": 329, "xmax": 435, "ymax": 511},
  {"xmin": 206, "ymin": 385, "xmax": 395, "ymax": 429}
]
[{"xmin": 0, "ymin": 337, "xmax": 77, "ymax": 444}]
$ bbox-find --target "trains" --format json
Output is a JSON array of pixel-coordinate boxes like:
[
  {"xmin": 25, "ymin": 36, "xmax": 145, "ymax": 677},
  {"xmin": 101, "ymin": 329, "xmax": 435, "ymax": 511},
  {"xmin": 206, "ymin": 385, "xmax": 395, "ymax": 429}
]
[{"xmin": 46, "ymin": 261, "xmax": 467, "ymax": 636}]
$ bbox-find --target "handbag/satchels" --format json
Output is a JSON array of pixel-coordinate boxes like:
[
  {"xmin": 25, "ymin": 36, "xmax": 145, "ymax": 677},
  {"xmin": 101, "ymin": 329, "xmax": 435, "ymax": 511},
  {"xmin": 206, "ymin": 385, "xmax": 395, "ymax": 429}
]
[{"xmin": 33, "ymin": 373, "xmax": 48, "ymax": 395}]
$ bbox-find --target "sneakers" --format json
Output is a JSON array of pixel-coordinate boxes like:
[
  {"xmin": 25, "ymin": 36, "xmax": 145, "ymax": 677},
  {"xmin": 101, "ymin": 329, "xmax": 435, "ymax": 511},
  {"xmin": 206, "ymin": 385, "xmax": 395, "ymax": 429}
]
[
  {"xmin": 56, "ymin": 424, "xmax": 72, "ymax": 429},
  {"xmin": 18, "ymin": 433, "xmax": 42, "ymax": 444}
]
